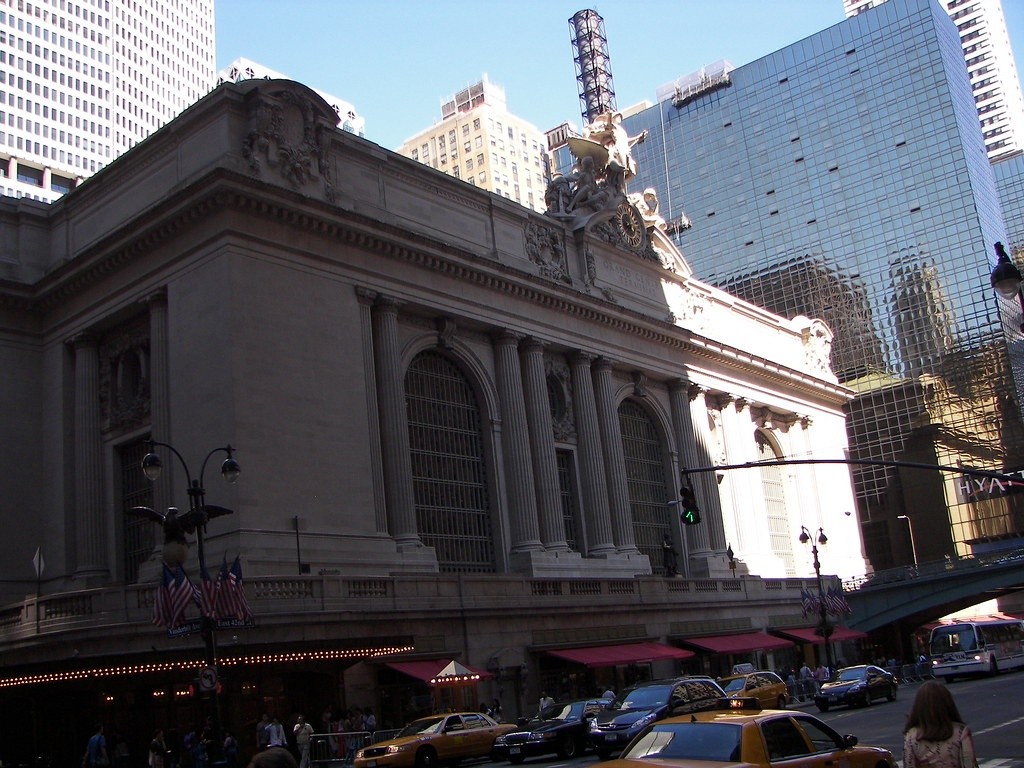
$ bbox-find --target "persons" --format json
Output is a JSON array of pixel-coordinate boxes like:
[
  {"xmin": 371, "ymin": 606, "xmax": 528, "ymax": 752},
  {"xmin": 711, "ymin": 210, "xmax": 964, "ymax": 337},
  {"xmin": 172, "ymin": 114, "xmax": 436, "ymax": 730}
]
[
  {"xmin": 633, "ymin": 187, "xmax": 666, "ymax": 228},
  {"xmin": 539, "ymin": 690, "xmax": 555, "ymax": 712},
  {"xmin": 566, "ymin": 156, "xmax": 596, "ymax": 212},
  {"xmin": 912, "ymin": 651, "xmax": 927, "ymax": 662},
  {"xmin": 82, "ymin": 707, "xmax": 376, "ymax": 768},
  {"xmin": 864, "ymin": 655, "xmax": 897, "ymax": 668},
  {"xmin": 662, "ymin": 533, "xmax": 681, "ymax": 575},
  {"xmin": 903, "ymin": 681, "xmax": 977, "ymax": 768},
  {"xmin": 775, "ymin": 661, "xmax": 830, "ymax": 681},
  {"xmin": 607, "ymin": 110, "xmax": 647, "ymax": 166},
  {"xmin": 479, "ymin": 698, "xmax": 503, "ymax": 722},
  {"xmin": 602, "ymin": 686, "xmax": 616, "ymax": 698}
]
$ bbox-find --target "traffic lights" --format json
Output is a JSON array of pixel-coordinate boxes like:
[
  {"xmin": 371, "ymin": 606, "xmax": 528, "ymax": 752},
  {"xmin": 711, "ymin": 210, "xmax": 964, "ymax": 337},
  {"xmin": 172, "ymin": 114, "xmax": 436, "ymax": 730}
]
[
  {"xmin": 680, "ymin": 485, "xmax": 701, "ymax": 525},
  {"xmin": 188, "ymin": 683, "xmax": 200, "ymax": 698}
]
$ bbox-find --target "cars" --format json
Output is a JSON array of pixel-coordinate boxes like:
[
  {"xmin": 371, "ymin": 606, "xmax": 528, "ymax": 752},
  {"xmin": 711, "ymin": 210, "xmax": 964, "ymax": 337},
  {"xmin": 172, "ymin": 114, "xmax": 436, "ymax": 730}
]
[
  {"xmin": 490, "ymin": 696, "xmax": 625, "ymax": 766},
  {"xmin": 813, "ymin": 664, "xmax": 899, "ymax": 713},
  {"xmin": 581, "ymin": 696, "xmax": 900, "ymax": 768},
  {"xmin": 352, "ymin": 710, "xmax": 519, "ymax": 768},
  {"xmin": 715, "ymin": 662, "xmax": 790, "ymax": 710}
]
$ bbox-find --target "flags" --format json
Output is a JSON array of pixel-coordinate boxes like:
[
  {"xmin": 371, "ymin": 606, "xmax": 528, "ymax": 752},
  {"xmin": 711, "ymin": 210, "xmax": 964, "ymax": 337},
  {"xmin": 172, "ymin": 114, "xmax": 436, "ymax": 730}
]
[
  {"xmin": 800, "ymin": 584, "xmax": 852, "ymax": 619},
  {"xmin": 150, "ymin": 556, "xmax": 253, "ymax": 630}
]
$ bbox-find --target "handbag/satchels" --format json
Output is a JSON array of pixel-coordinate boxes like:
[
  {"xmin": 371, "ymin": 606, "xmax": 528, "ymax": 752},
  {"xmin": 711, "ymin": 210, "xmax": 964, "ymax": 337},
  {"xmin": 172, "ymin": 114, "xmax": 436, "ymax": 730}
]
[{"xmin": 95, "ymin": 735, "xmax": 109, "ymax": 766}]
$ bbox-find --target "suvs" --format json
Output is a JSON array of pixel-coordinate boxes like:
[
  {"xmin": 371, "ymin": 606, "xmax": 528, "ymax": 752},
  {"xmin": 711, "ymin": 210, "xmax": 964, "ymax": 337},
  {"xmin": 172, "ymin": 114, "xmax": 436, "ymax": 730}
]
[{"xmin": 583, "ymin": 674, "xmax": 731, "ymax": 762}]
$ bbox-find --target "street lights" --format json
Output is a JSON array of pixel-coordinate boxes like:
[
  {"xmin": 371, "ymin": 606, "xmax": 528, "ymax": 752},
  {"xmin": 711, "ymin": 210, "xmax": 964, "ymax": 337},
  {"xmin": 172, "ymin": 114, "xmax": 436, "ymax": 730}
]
[
  {"xmin": 798, "ymin": 525, "xmax": 834, "ymax": 677},
  {"xmin": 140, "ymin": 437, "xmax": 244, "ymax": 768},
  {"xmin": 897, "ymin": 515, "xmax": 918, "ymax": 570}
]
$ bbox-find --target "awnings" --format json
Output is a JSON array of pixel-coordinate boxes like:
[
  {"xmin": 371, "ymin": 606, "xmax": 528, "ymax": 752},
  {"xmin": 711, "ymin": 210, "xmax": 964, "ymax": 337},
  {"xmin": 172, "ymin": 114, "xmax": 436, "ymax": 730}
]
[
  {"xmin": 683, "ymin": 633, "xmax": 794, "ymax": 654},
  {"xmin": 386, "ymin": 659, "xmax": 493, "ymax": 685},
  {"xmin": 546, "ymin": 642, "xmax": 695, "ymax": 668},
  {"xmin": 778, "ymin": 624, "xmax": 867, "ymax": 643}
]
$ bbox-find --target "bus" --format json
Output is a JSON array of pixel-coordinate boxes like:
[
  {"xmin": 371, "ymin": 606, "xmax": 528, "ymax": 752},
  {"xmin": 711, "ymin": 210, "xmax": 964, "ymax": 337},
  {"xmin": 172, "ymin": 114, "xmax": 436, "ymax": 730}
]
[{"xmin": 927, "ymin": 617, "xmax": 1024, "ymax": 684}]
